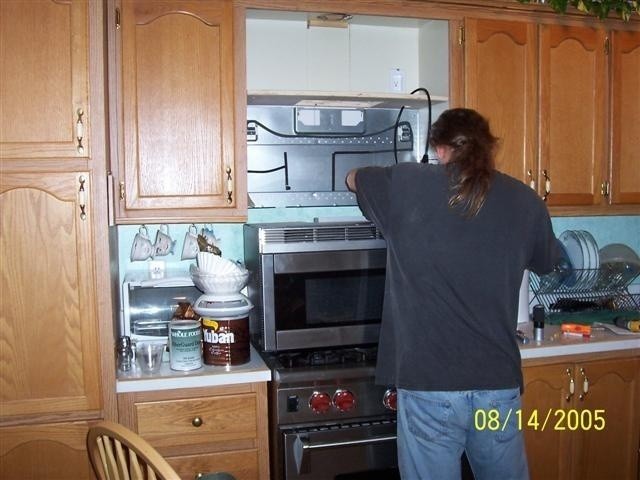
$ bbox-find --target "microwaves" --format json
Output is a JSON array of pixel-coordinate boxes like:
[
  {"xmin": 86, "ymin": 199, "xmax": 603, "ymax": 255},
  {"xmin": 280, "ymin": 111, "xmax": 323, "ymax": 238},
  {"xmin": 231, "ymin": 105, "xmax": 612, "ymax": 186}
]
[{"xmin": 120, "ymin": 275, "xmax": 248, "ymax": 355}]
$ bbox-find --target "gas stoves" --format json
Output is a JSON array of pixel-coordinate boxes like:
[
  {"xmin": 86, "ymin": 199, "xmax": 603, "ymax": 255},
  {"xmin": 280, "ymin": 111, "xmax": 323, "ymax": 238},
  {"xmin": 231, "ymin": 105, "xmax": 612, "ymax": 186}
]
[{"xmin": 258, "ymin": 343, "xmax": 400, "ymax": 430}]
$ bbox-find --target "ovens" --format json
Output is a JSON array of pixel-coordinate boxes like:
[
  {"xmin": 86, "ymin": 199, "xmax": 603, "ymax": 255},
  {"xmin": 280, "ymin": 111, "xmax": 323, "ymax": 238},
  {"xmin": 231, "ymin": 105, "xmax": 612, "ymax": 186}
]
[
  {"xmin": 268, "ymin": 427, "xmax": 402, "ymax": 480},
  {"xmin": 242, "ymin": 219, "xmax": 389, "ymax": 352}
]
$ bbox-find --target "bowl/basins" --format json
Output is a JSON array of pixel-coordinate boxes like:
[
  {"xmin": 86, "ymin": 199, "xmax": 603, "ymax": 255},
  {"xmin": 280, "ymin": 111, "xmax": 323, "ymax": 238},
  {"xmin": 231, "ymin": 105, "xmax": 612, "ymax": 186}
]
[
  {"xmin": 190, "ymin": 272, "xmax": 253, "ymax": 296},
  {"xmin": 599, "ymin": 244, "xmax": 639, "ymax": 269}
]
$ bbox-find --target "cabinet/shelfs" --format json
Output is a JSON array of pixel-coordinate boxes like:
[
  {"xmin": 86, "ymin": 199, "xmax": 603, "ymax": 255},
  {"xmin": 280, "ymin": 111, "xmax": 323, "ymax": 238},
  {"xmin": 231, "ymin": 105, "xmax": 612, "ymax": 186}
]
[
  {"xmin": 1, "ymin": 0, "xmax": 116, "ymax": 419},
  {"xmin": 116, "ymin": 1, "xmax": 250, "ymax": 224},
  {"xmin": 0, "ymin": 422, "xmax": 120, "ymax": 479},
  {"xmin": 116, "ymin": 334, "xmax": 277, "ymax": 478},
  {"xmin": 446, "ymin": 1, "xmax": 607, "ymax": 218},
  {"xmin": 520, "ymin": 350, "xmax": 640, "ymax": 480},
  {"xmin": 608, "ymin": 0, "xmax": 640, "ymax": 219}
]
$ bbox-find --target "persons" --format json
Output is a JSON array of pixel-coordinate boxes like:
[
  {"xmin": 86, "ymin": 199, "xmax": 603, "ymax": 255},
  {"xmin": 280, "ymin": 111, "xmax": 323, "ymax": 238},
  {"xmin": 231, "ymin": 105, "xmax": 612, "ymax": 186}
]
[{"xmin": 344, "ymin": 107, "xmax": 560, "ymax": 479}]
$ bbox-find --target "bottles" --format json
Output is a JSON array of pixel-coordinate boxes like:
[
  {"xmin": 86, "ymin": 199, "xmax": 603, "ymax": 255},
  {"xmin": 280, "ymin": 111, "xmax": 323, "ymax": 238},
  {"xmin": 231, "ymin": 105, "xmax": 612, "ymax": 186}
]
[
  {"xmin": 393, "ymin": 68, "xmax": 404, "ymax": 89},
  {"xmin": 118, "ymin": 334, "xmax": 132, "ymax": 372}
]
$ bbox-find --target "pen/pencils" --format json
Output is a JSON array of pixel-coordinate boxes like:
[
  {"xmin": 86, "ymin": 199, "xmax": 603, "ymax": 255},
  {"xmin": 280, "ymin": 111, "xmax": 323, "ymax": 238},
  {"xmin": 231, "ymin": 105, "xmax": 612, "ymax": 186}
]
[{"xmin": 564, "ymin": 332, "xmax": 596, "ymax": 338}]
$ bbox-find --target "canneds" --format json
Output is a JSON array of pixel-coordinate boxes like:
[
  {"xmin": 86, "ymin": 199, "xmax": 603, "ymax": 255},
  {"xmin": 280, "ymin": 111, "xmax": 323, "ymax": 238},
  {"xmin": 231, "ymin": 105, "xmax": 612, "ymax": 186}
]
[{"xmin": 168, "ymin": 320, "xmax": 202, "ymax": 372}]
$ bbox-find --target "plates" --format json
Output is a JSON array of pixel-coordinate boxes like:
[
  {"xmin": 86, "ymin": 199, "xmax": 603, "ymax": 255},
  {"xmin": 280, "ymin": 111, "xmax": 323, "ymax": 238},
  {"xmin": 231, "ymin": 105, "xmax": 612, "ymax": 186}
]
[{"xmin": 558, "ymin": 229, "xmax": 600, "ymax": 289}]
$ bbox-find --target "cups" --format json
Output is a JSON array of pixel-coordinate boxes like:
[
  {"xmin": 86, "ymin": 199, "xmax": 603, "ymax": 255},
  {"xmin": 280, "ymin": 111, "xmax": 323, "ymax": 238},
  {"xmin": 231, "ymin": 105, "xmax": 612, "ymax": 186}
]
[
  {"xmin": 130, "ymin": 225, "xmax": 220, "ymax": 262},
  {"xmin": 134, "ymin": 342, "xmax": 164, "ymax": 372}
]
